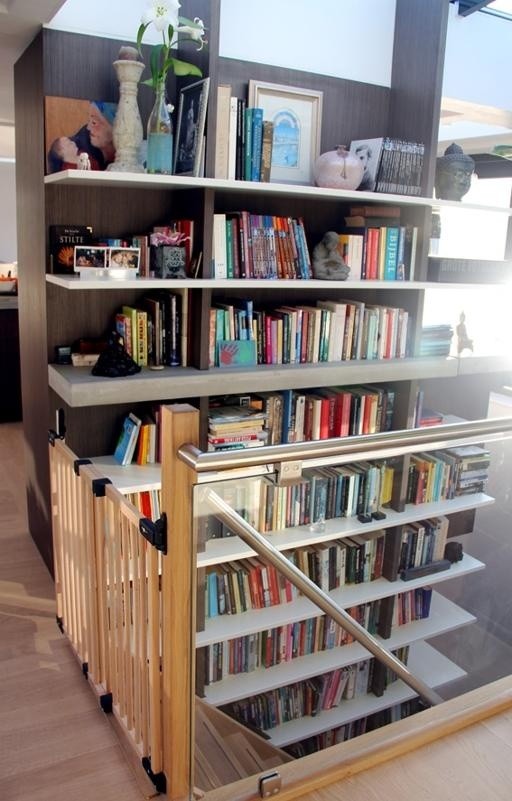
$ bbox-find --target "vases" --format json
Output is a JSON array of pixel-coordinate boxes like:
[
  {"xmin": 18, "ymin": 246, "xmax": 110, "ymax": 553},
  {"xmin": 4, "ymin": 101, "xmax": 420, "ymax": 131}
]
[
  {"xmin": 144, "ymin": 89, "xmax": 174, "ymax": 174},
  {"xmin": 313, "ymin": 144, "xmax": 366, "ymax": 191}
]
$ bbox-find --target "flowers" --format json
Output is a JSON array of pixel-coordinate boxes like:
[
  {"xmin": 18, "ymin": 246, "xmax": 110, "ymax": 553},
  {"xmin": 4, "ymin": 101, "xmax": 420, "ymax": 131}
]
[{"xmin": 132, "ymin": 1, "xmax": 210, "ymax": 134}]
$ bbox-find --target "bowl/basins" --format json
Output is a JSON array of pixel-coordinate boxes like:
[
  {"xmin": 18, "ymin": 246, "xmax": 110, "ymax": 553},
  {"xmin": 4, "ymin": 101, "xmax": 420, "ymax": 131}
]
[{"xmin": 0, "ymin": 280, "xmax": 16, "ymax": 294}]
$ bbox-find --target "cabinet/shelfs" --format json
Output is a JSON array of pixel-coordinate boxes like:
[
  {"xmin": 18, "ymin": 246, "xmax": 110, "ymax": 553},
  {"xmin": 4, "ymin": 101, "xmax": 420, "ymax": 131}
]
[
  {"xmin": 34, "ymin": 159, "xmax": 463, "ymax": 413},
  {"xmin": 81, "ymin": 411, "xmax": 497, "ymax": 759}
]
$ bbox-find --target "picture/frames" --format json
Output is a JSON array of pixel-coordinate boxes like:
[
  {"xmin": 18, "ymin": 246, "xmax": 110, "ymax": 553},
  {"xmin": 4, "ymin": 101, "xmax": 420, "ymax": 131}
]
[
  {"xmin": 244, "ymin": 78, "xmax": 325, "ymax": 188},
  {"xmin": 169, "ymin": 76, "xmax": 212, "ymax": 179}
]
